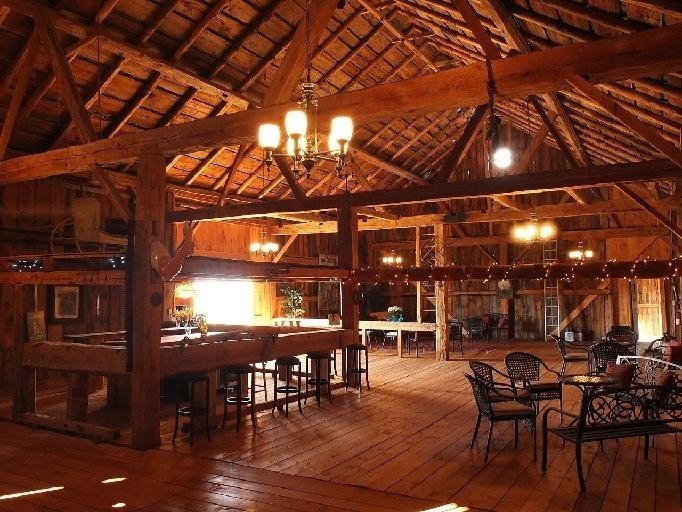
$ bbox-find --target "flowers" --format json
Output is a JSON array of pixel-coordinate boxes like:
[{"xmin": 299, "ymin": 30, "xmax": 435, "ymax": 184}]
[
  {"xmin": 173, "ymin": 305, "xmax": 205, "ymax": 328},
  {"xmin": 388, "ymin": 305, "xmax": 403, "ymax": 315}
]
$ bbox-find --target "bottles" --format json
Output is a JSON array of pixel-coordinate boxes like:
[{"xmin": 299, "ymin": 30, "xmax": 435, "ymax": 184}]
[{"xmin": 199, "ymin": 316, "xmax": 208, "ymax": 336}]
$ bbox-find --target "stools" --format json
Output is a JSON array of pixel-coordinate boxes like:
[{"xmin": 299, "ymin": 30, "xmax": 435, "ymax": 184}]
[
  {"xmin": 223, "ymin": 362, "xmax": 258, "ymax": 431},
  {"xmin": 343, "ymin": 342, "xmax": 371, "ymax": 391},
  {"xmin": 304, "ymin": 351, "xmax": 335, "ymax": 405},
  {"xmin": 172, "ymin": 370, "xmax": 213, "ymax": 447},
  {"xmin": 273, "ymin": 354, "xmax": 304, "ymax": 416}
]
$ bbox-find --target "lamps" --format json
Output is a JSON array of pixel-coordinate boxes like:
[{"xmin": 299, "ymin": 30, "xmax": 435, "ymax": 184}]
[
  {"xmin": 382, "ymin": 246, "xmax": 404, "ymax": 266},
  {"xmin": 248, "ymin": 215, "xmax": 281, "ymax": 256},
  {"xmin": 513, "ymin": 192, "xmax": 556, "ymax": 243},
  {"xmin": 257, "ymin": 0, "xmax": 354, "ymax": 184},
  {"xmin": 566, "ymin": 230, "xmax": 593, "ymax": 267}
]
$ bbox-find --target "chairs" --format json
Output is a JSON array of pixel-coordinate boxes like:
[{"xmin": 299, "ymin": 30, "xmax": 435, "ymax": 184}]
[
  {"xmin": 360, "ymin": 311, "xmax": 511, "ymax": 355},
  {"xmin": 460, "ymin": 322, "xmax": 681, "ymax": 493}
]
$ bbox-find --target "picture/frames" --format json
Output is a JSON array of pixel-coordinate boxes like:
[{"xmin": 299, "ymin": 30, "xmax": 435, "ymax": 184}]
[{"xmin": 46, "ymin": 284, "xmax": 85, "ymax": 325}]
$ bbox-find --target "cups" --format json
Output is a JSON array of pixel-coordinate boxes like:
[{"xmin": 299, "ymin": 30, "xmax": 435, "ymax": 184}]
[{"xmin": 274, "ymin": 320, "xmax": 302, "ymax": 327}]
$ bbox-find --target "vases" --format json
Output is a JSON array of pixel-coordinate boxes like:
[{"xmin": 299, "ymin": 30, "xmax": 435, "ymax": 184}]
[{"xmin": 184, "ymin": 324, "xmax": 193, "ymax": 334}]
